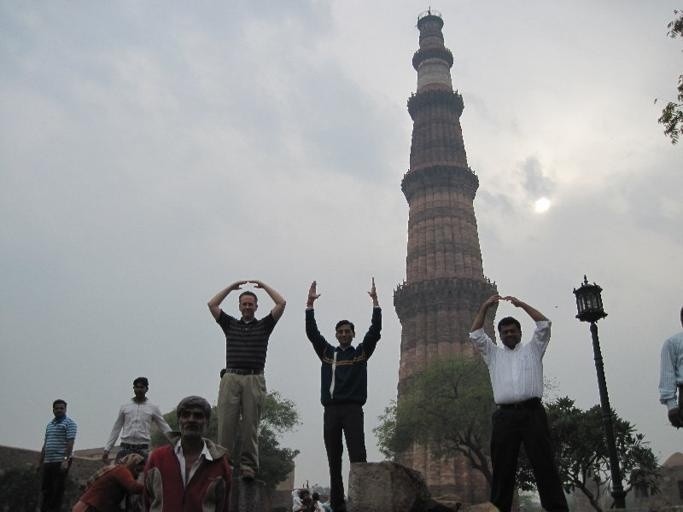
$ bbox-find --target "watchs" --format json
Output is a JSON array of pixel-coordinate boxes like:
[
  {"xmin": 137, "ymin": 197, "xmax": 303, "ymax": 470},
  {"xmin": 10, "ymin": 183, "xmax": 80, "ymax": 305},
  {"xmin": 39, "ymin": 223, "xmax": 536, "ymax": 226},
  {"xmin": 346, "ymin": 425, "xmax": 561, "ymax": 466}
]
[{"xmin": 63, "ymin": 456, "xmax": 70, "ymax": 460}]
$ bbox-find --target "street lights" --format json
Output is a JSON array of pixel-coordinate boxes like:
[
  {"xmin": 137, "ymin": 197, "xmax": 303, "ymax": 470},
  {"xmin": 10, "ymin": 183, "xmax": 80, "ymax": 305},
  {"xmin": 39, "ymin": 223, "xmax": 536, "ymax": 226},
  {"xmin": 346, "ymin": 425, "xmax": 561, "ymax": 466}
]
[{"xmin": 573, "ymin": 272, "xmax": 631, "ymax": 512}]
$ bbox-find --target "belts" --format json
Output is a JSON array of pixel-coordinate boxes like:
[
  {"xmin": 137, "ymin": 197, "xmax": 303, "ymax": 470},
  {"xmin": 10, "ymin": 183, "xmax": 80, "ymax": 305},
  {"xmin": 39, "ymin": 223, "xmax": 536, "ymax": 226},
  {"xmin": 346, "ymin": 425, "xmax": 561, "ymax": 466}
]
[
  {"xmin": 496, "ymin": 402, "xmax": 541, "ymax": 410},
  {"xmin": 220, "ymin": 368, "xmax": 263, "ymax": 377}
]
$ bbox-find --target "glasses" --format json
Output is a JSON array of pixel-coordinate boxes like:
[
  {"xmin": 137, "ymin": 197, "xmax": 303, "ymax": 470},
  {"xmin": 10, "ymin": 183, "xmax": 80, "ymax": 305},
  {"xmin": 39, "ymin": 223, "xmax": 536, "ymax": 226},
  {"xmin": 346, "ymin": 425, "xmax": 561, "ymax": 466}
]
[{"xmin": 179, "ymin": 410, "xmax": 204, "ymax": 420}]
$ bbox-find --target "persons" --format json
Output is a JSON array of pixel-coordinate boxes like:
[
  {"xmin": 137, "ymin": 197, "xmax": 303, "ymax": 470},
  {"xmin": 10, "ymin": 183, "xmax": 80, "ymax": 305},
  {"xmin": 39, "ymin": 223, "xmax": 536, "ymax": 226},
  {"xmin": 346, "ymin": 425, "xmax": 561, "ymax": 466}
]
[
  {"xmin": 658, "ymin": 308, "xmax": 683, "ymax": 431},
  {"xmin": 136, "ymin": 397, "xmax": 232, "ymax": 512},
  {"xmin": 297, "ymin": 489, "xmax": 316, "ymax": 512},
  {"xmin": 468, "ymin": 295, "xmax": 568, "ymax": 512},
  {"xmin": 305, "ymin": 277, "xmax": 381, "ymax": 512},
  {"xmin": 312, "ymin": 492, "xmax": 325, "ymax": 512},
  {"xmin": 103, "ymin": 377, "xmax": 176, "ymax": 512},
  {"xmin": 36, "ymin": 399, "xmax": 75, "ymax": 512},
  {"xmin": 207, "ymin": 280, "xmax": 286, "ymax": 481},
  {"xmin": 71, "ymin": 454, "xmax": 145, "ymax": 512}
]
